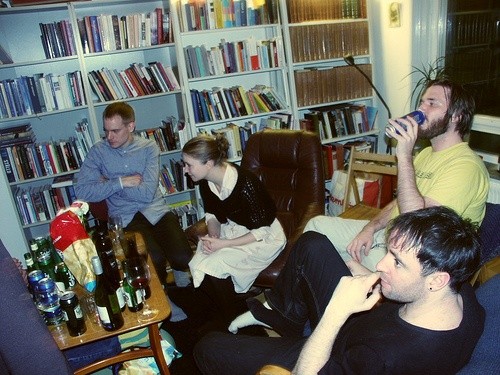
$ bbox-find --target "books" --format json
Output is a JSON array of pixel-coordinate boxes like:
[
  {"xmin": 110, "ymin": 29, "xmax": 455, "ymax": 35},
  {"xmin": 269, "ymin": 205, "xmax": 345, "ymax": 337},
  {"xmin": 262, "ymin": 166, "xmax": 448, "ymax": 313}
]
[
  {"xmin": 0, "ymin": 44, "xmax": 14, "ymax": 64},
  {"xmin": 190, "ymin": 84, "xmax": 294, "ymax": 159},
  {"xmin": 38, "ymin": 7, "xmax": 174, "ymax": 59},
  {"xmin": 87, "ymin": 61, "xmax": 182, "ymax": 102},
  {"xmin": 183, "ymin": 37, "xmax": 282, "ymax": 80},
  {"xmin": 0, "ymin": 69, "xmax": 86, "ymax": 121},
  {"xmin": 167, "ymin": 199, "xmax": 200, "ymax": 232},
  {"xmin": 287, "ymin": 0, "xmax": 378, "ymax": 179},
  {"xmin": 445, "ymin": 0, "xmax": 500, "ymax": 119},
  {"xmin": 0, "ymin": 119, "xmax": 95, "ymax": 228},
  {"xmin": 176, "ymin": 0, "xmax": 279, "ymax": 33},
  {"xmin": 133, "ymin": 115, "xmax": 195, "ymax": 196}
]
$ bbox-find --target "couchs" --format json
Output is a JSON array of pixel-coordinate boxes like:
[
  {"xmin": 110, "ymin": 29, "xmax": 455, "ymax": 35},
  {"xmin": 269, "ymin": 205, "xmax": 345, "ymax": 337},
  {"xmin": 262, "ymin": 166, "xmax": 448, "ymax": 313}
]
[{"xmin": 457, "ymin": 203, "xmax": 500, "ymax": 375}]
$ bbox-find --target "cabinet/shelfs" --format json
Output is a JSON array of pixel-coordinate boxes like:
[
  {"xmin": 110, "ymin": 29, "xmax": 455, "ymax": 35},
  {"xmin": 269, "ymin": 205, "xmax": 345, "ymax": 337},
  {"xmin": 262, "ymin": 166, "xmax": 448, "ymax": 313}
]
[{"xmin": 0, "ymin": 1, "xmax": 379, "ymax": 262}]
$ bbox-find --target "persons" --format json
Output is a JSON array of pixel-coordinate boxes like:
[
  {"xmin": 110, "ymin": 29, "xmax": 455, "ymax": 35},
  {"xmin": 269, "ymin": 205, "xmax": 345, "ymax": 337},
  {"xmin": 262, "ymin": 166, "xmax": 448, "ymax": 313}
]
[
  {"xmin": 228, "ymin": 76, "xmax": 491, "ymax": 335},
  {"xmin": 182, "ymin": 134, "xmax": 288, "ymax": 325},
  {"xmin": 13, "ymin": 258, "xmax": 30, "ymax": 287},
  {"xmin": 76, "ymin": 102, "xmax": 194, "ymax": 289},
  {"xmin": 159, "ymin": 205, "xmax": 485, "ymax": 375}
]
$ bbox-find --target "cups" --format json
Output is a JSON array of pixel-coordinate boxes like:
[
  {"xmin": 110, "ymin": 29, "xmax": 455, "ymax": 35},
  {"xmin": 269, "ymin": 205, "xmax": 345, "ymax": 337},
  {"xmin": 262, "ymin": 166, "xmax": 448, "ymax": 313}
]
[{"xmin": 120, "ymin": 231, "xmax": 138, "ymax": 255}]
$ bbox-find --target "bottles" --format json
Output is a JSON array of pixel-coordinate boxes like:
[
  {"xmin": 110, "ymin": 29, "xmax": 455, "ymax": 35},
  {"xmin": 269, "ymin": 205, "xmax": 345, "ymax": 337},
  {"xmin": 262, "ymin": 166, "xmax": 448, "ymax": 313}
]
[
  {"xmin": 124, "ymin": 240, "xmax": 151, "ymax": 300},
  {"xmin": 92, "ymin": 218, "xmax": 108, "ymax": 242},
  {"xmin": 97, "ymin": 251, "xmax": 126, "ymax": 312},
  {"xmin": 24, "ymin": 253, "xmax": 44, "ymax": 303},
  {"xmin": 28, "ymin": 239, "xmax": 42, "ymax": 270},
  {"xmin": 96, "ymin": 232, "xmax": 126, "ymax": 311},
  {"xmin": 92, "ymin": 257, "xmax": 124, "ymax": 331},
  {"xmin": 122, "ymin": 260, "xmax": 143, "ymax": 313}
]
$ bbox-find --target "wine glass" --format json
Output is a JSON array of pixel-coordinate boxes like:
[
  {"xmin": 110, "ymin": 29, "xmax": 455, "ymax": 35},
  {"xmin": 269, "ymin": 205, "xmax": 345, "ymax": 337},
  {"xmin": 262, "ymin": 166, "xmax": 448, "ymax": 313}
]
[{"xmin": 108, "ymin": 215, "xmax": 124, "ymax": 246}]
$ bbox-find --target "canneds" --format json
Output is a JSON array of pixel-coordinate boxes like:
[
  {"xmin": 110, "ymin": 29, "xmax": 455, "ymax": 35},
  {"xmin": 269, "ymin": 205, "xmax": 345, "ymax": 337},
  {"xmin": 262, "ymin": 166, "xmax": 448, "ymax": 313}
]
[
  {"xmin": 59, "ymin": 290, "xmax": 87, "ymax": 337},
  {"xmin": 384, "ymin": 110, "xmax": 426, "ymax": 138},
  {"xmin": 34, "ymin": 235, "xmax": 76, "ymax": 289},
  {"xmin": 27, "ymin": 271, "xmax": 63, "ymax": 323}
]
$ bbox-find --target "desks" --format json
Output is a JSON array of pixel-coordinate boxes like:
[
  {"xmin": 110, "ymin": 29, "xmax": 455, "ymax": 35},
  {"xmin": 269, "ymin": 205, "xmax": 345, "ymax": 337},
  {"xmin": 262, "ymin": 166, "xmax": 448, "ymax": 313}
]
[{"xmin": 44, "ymin": 232, "xmax": 172, "ymax": 375}]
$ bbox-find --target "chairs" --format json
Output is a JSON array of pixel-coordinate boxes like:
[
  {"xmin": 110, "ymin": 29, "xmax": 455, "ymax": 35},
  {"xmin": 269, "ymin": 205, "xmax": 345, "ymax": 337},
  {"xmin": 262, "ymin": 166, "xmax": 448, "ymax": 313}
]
[
  {"xmin": 334, "ymin": 144, "xmax": 398, "ymax": 223},
  {"xmin": 188, "ymin": 129, "xmax": 323, "ymax": 293}
]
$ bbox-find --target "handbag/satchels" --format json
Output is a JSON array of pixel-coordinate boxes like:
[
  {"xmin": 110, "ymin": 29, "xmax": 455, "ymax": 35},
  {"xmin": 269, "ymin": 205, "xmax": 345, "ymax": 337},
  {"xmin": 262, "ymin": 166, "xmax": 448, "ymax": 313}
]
[{"xmin": 328, "ymin": 170, "xmax": 397, "ymax": 217}]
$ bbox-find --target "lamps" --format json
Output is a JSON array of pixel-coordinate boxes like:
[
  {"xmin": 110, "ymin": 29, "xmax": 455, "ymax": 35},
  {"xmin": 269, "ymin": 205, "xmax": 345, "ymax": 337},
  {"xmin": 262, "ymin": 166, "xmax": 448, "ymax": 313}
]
[{"xmin": 344, "ymin": 55, "xmax": 391, "ymax": 120}]
